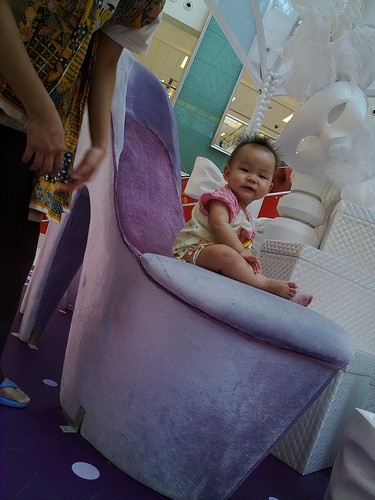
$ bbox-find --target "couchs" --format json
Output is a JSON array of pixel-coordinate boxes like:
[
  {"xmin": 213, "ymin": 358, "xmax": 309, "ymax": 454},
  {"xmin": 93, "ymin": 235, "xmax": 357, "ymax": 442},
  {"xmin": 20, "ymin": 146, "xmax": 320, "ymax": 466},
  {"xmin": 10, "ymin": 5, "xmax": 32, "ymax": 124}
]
[{"xmin": 58, "ymin": 49, "xmax": 354, "ymax": 500}]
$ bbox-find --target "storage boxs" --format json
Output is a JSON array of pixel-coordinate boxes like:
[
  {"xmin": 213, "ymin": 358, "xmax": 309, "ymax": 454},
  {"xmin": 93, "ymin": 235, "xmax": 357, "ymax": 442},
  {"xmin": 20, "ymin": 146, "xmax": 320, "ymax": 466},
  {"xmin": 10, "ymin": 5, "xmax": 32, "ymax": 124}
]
[
  {"xmin": 259, "ymin": 238, "xmax": 375, "ymax": 346},
  {"xmin": 269, "ymin": 345, "xmax": 375, "ymax": 476},
  {"xmin": 319, "ymin": 200, "xmax": 375, "ymax": 272}
]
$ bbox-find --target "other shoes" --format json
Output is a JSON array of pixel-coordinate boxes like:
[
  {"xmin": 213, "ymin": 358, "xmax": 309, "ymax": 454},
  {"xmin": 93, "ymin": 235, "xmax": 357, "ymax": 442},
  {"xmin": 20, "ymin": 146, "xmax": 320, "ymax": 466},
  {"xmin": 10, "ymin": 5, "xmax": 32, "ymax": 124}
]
[{"xmin": 0, "ymin": 377, "xmax": 30, "ymax": 408}]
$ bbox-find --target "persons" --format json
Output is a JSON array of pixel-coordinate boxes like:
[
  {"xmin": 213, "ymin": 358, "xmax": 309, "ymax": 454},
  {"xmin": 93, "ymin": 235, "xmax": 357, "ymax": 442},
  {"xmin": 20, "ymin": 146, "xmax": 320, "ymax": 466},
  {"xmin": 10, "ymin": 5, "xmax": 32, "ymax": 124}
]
[
  {"xmin": 0, "ymin": 0, "xmax": 170, "ymax": 407},
  {"xmin": 172, "ymin": 136, "xmax": 313, "ymax": 307}
]
